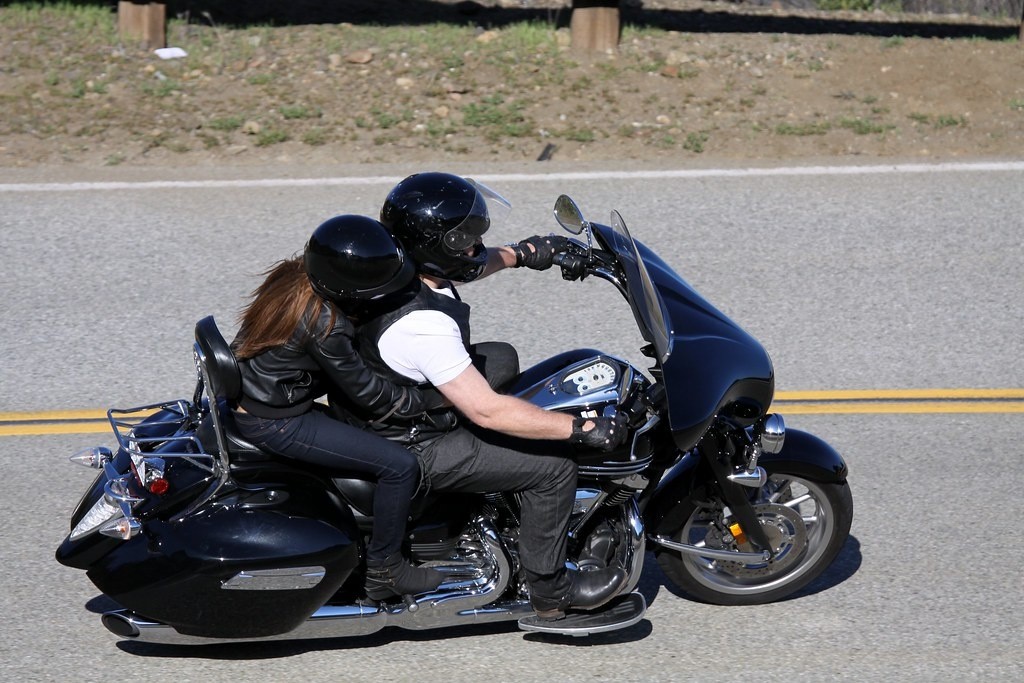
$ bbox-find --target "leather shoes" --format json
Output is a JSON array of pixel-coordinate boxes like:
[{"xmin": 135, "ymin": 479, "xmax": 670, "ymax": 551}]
[
  {"xmin": 524, "ymin": 567, "xmax": 628, "ymax": 622},
  {"xmin": 364, "ymin": 553, "xmax": 447, "ymax": 601}
]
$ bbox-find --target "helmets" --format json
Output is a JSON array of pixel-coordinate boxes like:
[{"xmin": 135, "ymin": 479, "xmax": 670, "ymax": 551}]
[
  {"xmin": 303, "ymin": 216, "xmax": 416, "ymax": 316},
  {"xmin": 379, "ymin": 173, "xmax": 490, "ymax": 283}
]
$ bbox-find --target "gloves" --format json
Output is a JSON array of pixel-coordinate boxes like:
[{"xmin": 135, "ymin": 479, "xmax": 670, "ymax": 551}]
[
  {"xmin": 567, "ymin": 414, "xmax": 627, "ymax": 452},
  {"xmin": 512, "ymin": 235, "xmax": 568, "ymax": 271}
]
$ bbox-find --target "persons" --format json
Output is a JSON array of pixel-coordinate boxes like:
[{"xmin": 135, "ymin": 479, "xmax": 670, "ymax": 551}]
[
  {"xmin": 326, "ymin": 172, "xmax": 628, "ymax": 623},
  {"xmin": 229, "ymin": 214, "xmax": 454, "ymax": 602}
]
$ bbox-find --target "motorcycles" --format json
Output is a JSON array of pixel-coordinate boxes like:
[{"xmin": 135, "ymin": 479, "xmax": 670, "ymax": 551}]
[{"xmin": 54, "ymin": 195, "xmax": 854, "ymax": 644}]
